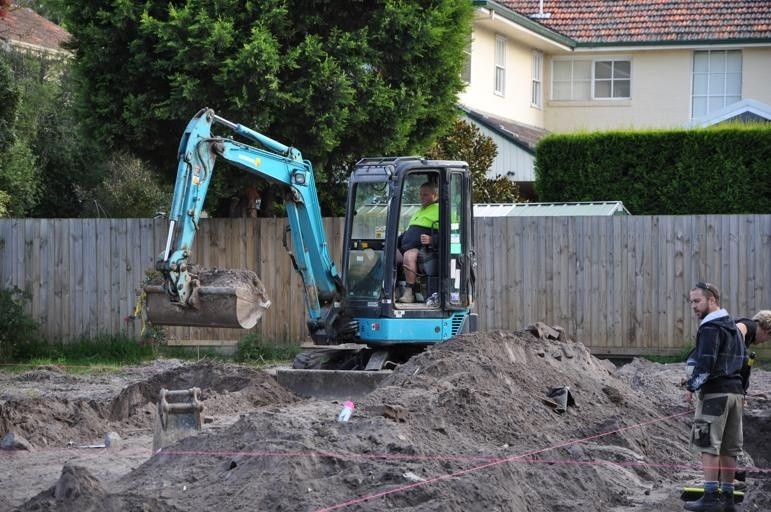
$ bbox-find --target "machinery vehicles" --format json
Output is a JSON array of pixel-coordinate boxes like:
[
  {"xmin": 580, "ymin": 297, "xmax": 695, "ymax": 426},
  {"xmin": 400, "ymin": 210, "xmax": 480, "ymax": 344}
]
[{"xmin": 143, "ymin": 106, "xmax": 477, "ymax": 371}]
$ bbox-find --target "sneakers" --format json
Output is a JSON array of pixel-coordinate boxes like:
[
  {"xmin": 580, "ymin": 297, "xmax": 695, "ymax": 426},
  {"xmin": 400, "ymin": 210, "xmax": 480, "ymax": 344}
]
[{"xmin": 394, "ymin": 286, "xmax": 414, "ymax": 303}]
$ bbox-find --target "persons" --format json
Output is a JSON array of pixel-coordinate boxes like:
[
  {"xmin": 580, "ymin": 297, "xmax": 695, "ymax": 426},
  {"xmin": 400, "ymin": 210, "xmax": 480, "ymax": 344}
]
[
  {"xmin": 395, "ymin": 182, "xmax": 457, "ymax": 303},
  {"xmin": 683, "ymin": 282, "xmax": 771, "ymax": 511}
]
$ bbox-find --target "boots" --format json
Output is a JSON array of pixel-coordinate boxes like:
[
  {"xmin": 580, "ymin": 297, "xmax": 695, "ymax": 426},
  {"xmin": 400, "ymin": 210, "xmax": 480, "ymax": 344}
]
[{"xmin": 683, "ymin": 491, "xmax": 736, "ymax": 511}]
